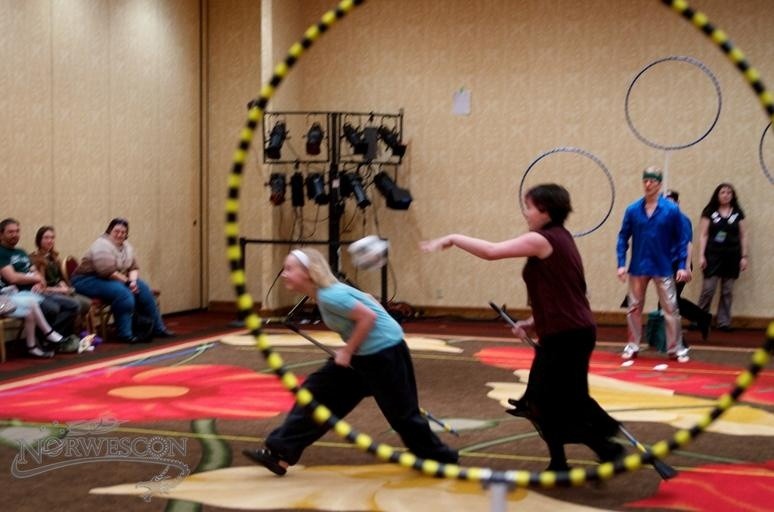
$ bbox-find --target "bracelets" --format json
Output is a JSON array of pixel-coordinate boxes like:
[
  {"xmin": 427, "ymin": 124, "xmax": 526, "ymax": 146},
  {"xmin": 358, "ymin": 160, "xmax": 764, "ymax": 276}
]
[
  {"xmin": 125, "ymin": 279, "xmax": 132, "ymax": 287},
  {"xmin": 740, "ymin": 255, "xmax": 747, "ymax": 258}
]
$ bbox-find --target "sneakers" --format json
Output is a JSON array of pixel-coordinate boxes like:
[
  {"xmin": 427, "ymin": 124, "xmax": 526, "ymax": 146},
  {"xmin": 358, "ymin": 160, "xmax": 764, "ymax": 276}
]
[
  {"xmin": 669, "ymin": 348, "xmax": 690, "ymax": 364},
  {"xmin": 78, "ymin": 324, "xmax": 179, "ymax": 346},
  {"xmin": 528, "ymin": 440, "xmax": 628, "ymax": 489},
  {"xmin": 618, "ymin": 342, "xmax": 639, "ymax": 361},
  {"xmin": 698, "ymin": 312, "xmax": 713, "ymax": 342}
]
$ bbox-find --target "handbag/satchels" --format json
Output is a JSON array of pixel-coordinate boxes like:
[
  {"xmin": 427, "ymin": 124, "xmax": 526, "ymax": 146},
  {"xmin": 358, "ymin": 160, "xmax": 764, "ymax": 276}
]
[{"xmin": 0, "ymin": 294, "xmax": 17, "ymax": 315}]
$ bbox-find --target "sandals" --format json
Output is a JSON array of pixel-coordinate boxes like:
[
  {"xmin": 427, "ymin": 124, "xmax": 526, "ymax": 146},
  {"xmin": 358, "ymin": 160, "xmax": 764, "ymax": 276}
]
[{"xmin": 241, "ymin": 446, "xmax": 289, "ymax": 478}]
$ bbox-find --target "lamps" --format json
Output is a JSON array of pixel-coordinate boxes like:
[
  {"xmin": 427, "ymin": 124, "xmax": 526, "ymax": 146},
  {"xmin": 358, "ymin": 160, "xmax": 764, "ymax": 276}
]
[{"xmin": 266, "ymin": 121, "xmax": 411, "ymax": 211}]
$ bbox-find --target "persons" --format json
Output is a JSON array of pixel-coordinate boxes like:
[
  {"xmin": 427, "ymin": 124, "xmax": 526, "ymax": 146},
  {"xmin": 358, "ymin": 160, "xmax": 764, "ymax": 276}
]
[
  {"xmin": 662, "ymin": 190, "xmax": 712, "ymax": 348},
  {"xmin": 70, "ymin": 217, "xmax": 177, "ymax": 344},
  {"xmin": 416, "ymin": 182, "xmax": 625, "ymax": 471},
  {"xmin": 0, "ymin": 218, "xmax": 70, "ymax": 359},
  {"xmin": 28, "ymin": 225, "xmax": 104, "ymax": 347},
  {"xmin": 697, "ymin": 182, "xmax": 748, "ymax": 330},
  {"xmin": 242, "ymin": 246, "xmax": 458, "ymax": 481},
  {"xmin": 616, "ymin": 166, "xmax": 692, "ymax": 364}
]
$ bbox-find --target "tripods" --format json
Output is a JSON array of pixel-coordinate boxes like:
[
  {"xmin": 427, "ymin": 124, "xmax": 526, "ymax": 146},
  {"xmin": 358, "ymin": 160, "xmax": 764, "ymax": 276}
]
[{"xmin": 284, "ymin": 110, "xmax": 362, "ymax": 320}]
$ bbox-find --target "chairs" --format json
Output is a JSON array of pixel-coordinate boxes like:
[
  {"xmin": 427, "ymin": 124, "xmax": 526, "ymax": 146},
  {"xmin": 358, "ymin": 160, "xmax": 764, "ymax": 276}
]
[{"xmin": 0, "ymin": 255, "xmax": 161, "ymax": 365}]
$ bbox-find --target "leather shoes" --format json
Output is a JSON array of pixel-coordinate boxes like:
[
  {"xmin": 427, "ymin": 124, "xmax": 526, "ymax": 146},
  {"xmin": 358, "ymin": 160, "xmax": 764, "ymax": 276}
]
[
  {"xmin": 27, "ymin": 342, "xmax": 56, "ymax": 359},
  {"xmin": 42, "ymin": 327, "xmax": 72, "ymax": 345}
]
